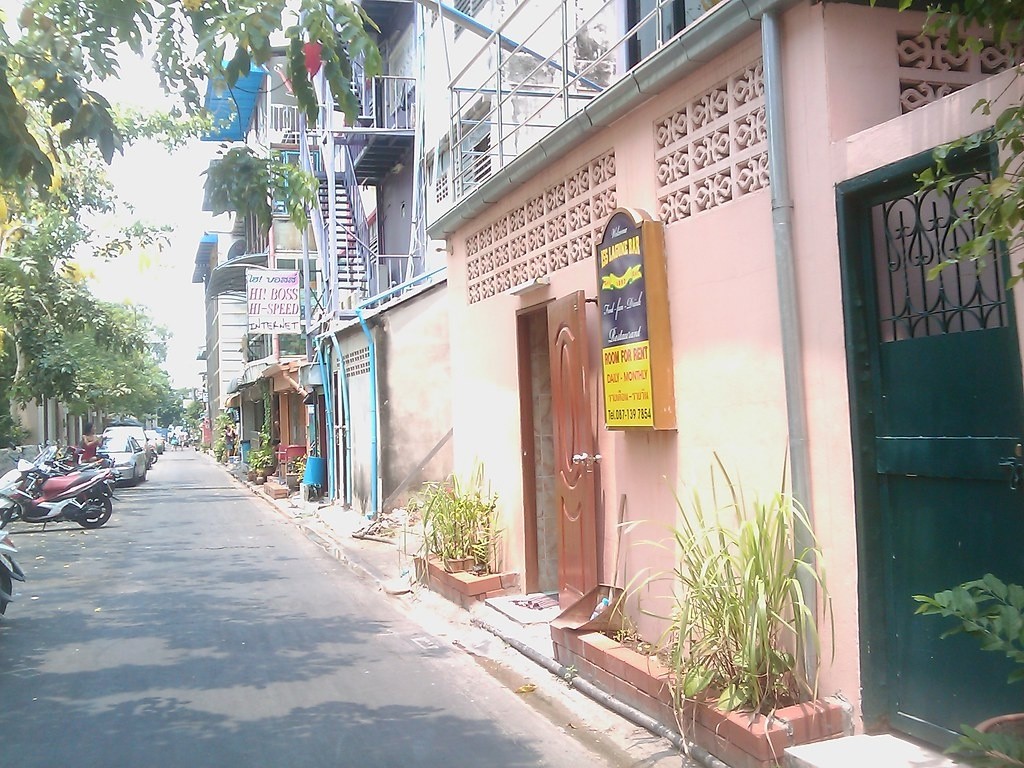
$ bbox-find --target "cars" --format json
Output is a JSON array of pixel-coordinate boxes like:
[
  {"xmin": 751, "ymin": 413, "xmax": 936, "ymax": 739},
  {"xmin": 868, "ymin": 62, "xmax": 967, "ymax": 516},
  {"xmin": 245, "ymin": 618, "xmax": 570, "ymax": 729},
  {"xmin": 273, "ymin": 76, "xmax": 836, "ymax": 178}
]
[{"xmin": 97, "ymin": 427, "xmax": 166, "ymax": 487}]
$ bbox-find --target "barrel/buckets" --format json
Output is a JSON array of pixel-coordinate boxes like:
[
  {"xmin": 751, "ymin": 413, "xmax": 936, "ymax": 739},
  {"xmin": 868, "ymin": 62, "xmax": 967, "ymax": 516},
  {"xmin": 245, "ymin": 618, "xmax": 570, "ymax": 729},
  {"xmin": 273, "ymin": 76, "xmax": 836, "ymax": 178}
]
[{"xmin": 302, "ymin": 456, "xmax": 326, "ymax": 488}]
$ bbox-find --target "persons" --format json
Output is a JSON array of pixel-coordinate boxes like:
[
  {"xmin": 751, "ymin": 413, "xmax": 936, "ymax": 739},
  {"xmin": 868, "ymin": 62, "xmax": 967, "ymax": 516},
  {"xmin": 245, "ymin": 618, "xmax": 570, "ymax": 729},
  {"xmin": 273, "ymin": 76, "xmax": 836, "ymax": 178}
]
[
  {"xmin": 74, "ymin": 422, "xmax": 108, "ymax": 460},
  {"xmin": 178, "ymin": 430, "xmax": 186, "ymax": 451},
  {"xmin": 170, "ymin": 433, "xmax": 178, "ymax": 452},
  {"xmin": 224, "ymin": 424, "xmax": 237, "ymax": 458}
]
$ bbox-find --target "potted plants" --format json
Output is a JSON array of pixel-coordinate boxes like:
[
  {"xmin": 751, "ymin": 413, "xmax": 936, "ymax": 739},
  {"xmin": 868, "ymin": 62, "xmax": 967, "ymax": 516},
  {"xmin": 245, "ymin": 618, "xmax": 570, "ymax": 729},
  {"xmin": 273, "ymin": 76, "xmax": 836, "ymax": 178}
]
[
  {"xmin": 288, "ymin": 454, "xmax": 307, "ymax": 490},
  {"xmin": 214, "ymin": 441, "xmax": 224, "ymax": 456},
  {"xmin": 250, "ymin": 451, "xmax": 274, "ymax": 483}
]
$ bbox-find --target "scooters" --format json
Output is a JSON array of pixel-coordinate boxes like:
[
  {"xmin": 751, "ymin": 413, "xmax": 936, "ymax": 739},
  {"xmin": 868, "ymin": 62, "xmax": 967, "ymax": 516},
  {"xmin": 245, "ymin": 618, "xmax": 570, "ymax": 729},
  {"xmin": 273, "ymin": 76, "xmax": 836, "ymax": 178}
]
[
  {"xmin": 0, "ymin": 529, "xmax": 25, "ymax": 618},
  {"xmin": 0, "ymin": 439, "xmax": 120, "ymax": 531}
]
